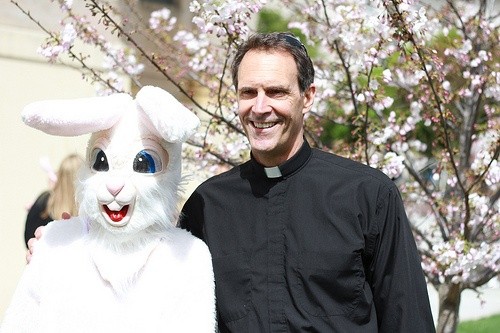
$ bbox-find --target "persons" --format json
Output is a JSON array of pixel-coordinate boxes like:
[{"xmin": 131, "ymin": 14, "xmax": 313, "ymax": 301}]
[
  {"xmin": 23, "ymin": 155, "xmax": 83, "ymax": 251},
  {"xmin": 176, "ymin": 32, "xmax": 439, "ymax": 333}
]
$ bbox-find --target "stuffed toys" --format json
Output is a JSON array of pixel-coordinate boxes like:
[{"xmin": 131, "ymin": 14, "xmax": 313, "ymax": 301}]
[{"xmin": 2, "ymin": 85, "xmax": 220, "ymax": 333}]
